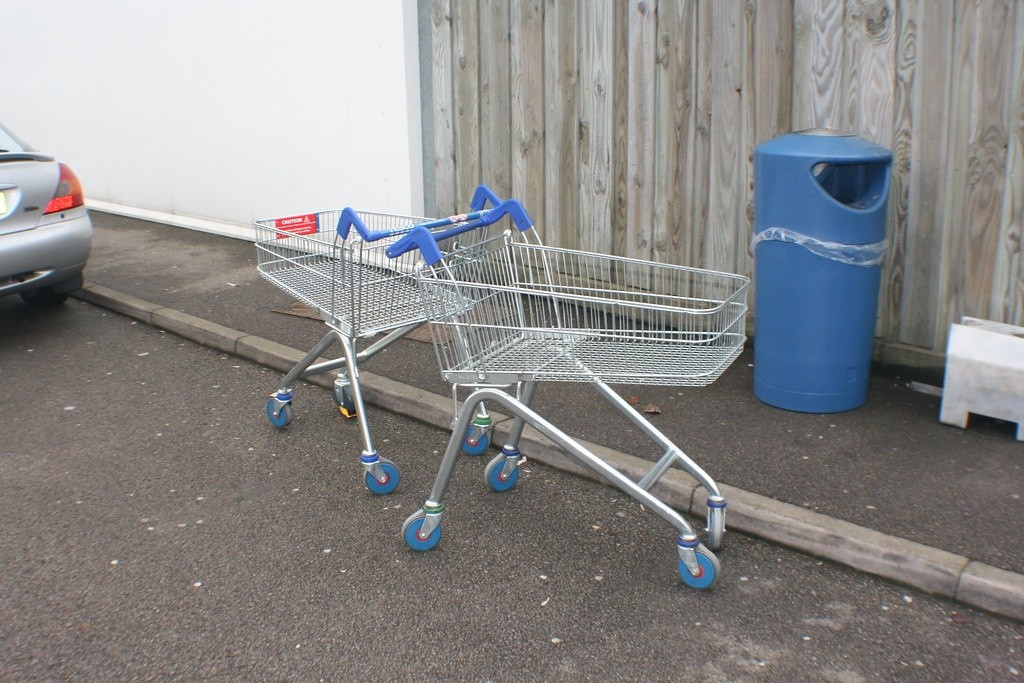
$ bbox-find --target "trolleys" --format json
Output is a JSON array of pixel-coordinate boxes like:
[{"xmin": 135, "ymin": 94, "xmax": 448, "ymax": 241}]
[
  {"xmin": 385, "ymin": 199, "xmax": 750, "ymax": 591},
  {"xmin": 254, "ymin": 185, "xmax": 505, "ymax": 492}
]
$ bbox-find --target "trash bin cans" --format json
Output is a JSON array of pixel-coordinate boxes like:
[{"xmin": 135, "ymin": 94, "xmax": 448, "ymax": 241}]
[{"xmin": 751, "ymin": 132, "xmax": 896, "ymax": 417}]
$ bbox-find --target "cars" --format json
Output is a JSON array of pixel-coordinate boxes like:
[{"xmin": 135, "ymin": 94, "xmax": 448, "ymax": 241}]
[{"xmin": 0, "ymin": 124, "xmax": 92, "ymax": 306}]
